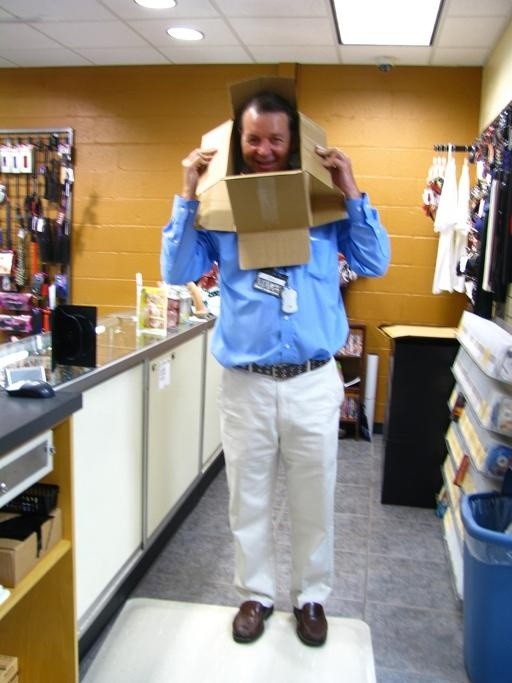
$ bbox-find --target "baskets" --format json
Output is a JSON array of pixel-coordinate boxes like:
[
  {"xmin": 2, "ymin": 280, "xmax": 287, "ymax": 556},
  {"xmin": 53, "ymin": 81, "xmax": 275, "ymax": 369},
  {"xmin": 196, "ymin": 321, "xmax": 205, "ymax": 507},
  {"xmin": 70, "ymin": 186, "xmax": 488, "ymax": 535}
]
[{"xmin": 0, "ymin": 482, "xmax": 59, "ymax": 513}]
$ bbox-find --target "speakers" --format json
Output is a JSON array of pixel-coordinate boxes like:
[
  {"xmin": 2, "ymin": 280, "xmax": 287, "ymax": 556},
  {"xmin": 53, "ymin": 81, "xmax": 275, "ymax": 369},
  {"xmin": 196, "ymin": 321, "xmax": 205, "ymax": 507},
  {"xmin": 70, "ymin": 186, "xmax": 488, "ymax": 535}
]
[{"xmin": 51, "ymin": 304, "xmax": 97, "ymax": 369}]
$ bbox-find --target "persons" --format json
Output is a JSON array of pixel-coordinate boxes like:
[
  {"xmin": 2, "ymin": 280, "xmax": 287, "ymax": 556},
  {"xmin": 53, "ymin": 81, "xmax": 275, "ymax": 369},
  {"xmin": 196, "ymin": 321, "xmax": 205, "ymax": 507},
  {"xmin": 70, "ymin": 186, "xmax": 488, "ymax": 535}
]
[{"xmin": 160, "ymin": 85, "xmax": 392, "ymax": 647}]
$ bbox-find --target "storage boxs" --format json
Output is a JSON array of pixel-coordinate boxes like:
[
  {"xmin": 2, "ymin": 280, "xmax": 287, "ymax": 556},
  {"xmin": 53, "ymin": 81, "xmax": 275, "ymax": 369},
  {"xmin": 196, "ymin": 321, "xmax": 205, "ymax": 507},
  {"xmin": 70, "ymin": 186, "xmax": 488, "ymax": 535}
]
[{"xmin": 191, "ymin": 73, "xmax": 350, "ymax": 272}]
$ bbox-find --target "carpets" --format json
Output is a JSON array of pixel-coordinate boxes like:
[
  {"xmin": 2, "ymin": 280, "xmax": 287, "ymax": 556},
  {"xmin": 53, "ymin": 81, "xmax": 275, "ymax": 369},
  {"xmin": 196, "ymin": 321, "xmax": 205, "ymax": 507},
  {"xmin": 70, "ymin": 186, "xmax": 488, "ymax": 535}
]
[{"xmin": 82, "ymin": 598, "xmax": 377, "ymax": 682}]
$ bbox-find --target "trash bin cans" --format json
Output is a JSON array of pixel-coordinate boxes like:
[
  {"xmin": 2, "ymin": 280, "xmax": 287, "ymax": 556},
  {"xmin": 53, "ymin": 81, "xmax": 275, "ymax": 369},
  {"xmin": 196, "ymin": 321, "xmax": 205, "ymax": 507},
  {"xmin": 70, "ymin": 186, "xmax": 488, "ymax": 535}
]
[{"xmin": 460, "ymin": 492, "xmax": 512, "ymax": 683}]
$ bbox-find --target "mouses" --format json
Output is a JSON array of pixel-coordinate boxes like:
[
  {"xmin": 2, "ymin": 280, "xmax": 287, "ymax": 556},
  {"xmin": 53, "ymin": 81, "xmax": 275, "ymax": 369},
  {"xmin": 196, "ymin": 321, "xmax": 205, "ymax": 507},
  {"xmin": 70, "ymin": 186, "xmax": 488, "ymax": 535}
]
[{"xmin": 5, "ymin": 378, "xmax": 56, "ymax": 398}]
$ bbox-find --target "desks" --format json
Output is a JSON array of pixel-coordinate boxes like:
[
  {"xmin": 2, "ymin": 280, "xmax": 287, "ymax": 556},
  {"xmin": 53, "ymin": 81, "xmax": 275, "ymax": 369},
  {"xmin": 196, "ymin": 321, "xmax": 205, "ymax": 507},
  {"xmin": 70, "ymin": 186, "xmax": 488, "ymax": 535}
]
[{"xmin": 378, "ymin": 319, "xmax": 463, "ymax": 509}]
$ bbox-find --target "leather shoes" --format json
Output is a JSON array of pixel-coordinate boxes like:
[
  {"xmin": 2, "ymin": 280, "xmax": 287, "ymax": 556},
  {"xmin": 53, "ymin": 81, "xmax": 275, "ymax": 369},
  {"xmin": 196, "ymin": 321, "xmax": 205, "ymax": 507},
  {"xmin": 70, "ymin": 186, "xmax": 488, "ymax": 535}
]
[
  {"xmin": 233, "ymin": 600, "xmax": 273, "ymax": 643},
  {"xmin": 293, "ymin": 602, "xmax": 327, "ymax": 646}
]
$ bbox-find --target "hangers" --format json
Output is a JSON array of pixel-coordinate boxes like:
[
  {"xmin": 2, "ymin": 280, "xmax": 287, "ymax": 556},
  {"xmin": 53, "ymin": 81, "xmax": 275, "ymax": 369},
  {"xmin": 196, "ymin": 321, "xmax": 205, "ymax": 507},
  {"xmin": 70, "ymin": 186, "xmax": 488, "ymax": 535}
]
[{"xmin": 430, "ymin": 140, "xmax": 479, "ymax": 181}]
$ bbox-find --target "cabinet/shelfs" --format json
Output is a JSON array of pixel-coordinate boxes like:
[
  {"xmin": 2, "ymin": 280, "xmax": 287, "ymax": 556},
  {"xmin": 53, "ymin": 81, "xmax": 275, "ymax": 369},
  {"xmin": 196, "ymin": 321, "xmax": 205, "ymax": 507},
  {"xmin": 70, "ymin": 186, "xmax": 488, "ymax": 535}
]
[
  {"xmin": 333, "ymin": 321, "xmax": 370, "ymax": 441},
  {"xmin": 1, "ymin": 410, "xmax": 80, "ymax": 683}
]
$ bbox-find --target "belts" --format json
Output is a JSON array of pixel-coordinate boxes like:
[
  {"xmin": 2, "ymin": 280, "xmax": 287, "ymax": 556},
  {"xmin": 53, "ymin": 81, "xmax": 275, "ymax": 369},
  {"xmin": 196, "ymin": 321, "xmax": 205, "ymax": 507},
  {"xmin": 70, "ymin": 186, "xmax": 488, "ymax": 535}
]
[{"xmin": 236, "ymin": 356, "xmax": 330, "ymax": 379}]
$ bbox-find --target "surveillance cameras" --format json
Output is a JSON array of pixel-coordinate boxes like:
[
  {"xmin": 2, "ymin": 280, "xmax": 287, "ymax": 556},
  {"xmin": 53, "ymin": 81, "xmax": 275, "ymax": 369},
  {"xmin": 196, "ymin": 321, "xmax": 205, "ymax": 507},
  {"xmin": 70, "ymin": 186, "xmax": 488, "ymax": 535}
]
[{"xmin": 377, "ymin": 56, "xmax": 396, "ymax": 73}]
